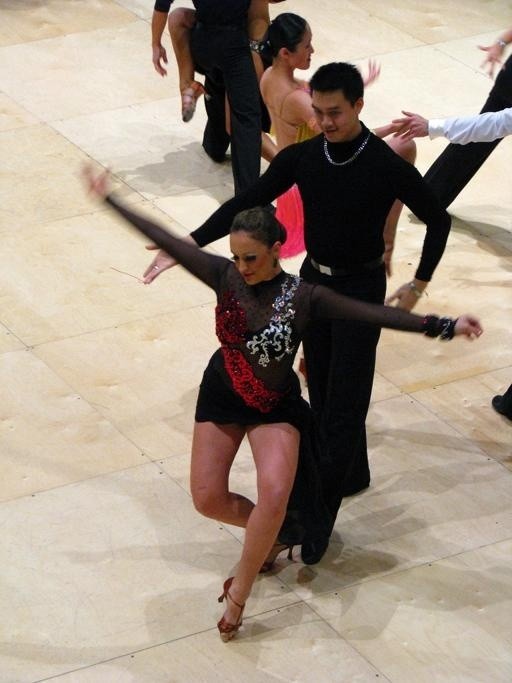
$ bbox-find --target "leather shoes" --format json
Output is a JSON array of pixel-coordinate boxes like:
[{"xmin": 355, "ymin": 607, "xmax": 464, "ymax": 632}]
[
  {"xmin": 492, "ymin": 395, "xmax": 504, "ymax": 413},
  {"xmin": 300, "ymin": 537, "xmax": 329, "ymax": 564}
]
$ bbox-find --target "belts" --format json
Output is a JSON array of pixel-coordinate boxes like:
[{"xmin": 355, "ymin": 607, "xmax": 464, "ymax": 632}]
[{"xmin": 307, "ymin": 257, "xmax": 384, "ymax": 277}]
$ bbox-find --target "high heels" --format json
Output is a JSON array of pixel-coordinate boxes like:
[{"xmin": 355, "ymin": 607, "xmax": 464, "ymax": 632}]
[
  {"xmin": 259, "ymin": 540, "xmax": 296, "ymax": 574},
  {"xmin": 218, "ymin": 576, "xmax": 246, "ymax": 643},
  {"xmin": 181, "ymin": 82, "xmax": 211, "ymax": 122}
]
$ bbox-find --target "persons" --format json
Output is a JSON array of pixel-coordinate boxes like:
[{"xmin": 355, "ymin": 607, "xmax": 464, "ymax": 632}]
[
  {"xmin": 258, "ymin": 12, "xmax": 417, "ymax": 278},
  {"xmin": 392, "ymin": 106, "xmax": 512, "ymax": 421},
  {"xmin": 149, "ymin": 0, "xmax": 272, "ymax": 197},
  {"xmin": 167, "ymin": 8, "xmax": 278, "ymax": 163},
  {"xmin": 141, "ymin": 62, "xmax": 452, "ymax": 496},
  {"xmin": 422, "ymin": 28, "xmax": 512, "ymax": 208},
  {"xmin": 80, "ymin": 160, "xmax": 484, "ymax": 643}
]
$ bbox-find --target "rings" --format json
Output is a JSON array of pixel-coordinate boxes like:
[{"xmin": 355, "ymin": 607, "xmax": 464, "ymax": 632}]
[{"xmin": 154, "ymin": 266, "xmax": 159, "ymax": 270}]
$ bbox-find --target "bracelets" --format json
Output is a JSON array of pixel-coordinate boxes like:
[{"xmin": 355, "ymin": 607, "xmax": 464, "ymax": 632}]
[
  {"xmin": 409, "ymin": 281, "xmax": 421, "ymax": 297},
  {"xmin": 495, "ymin": 38, "xmax": 506, "ymax": 48}
]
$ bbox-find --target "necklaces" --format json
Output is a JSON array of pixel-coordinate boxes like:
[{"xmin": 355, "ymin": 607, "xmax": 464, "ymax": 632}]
[{"xmin": 323, "ymin": 131, "xmax": 371, "ymax": 166}]
